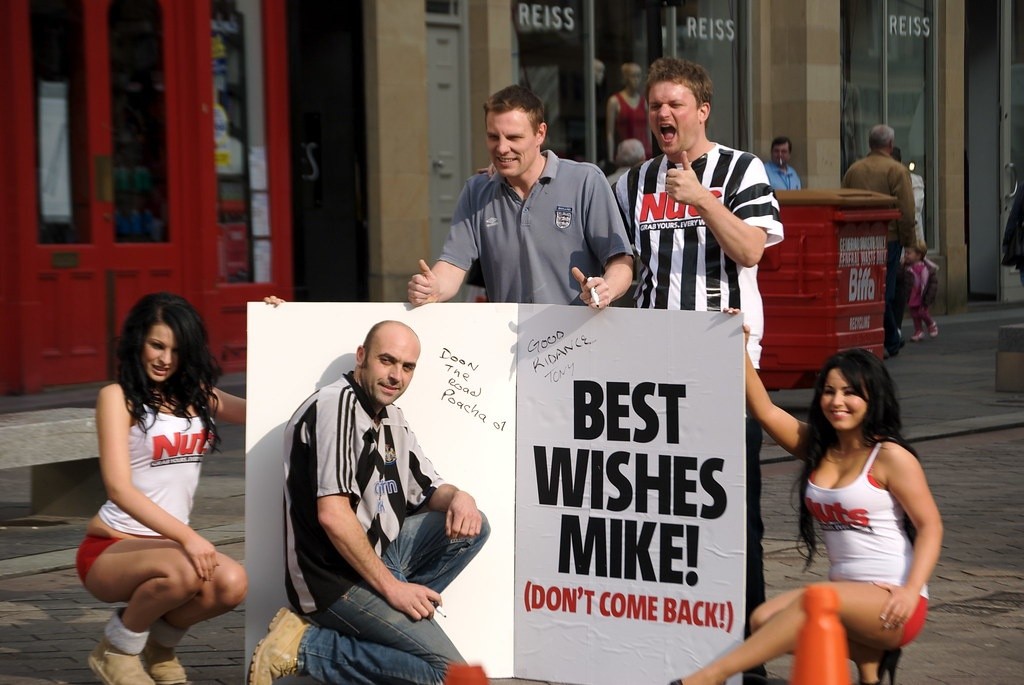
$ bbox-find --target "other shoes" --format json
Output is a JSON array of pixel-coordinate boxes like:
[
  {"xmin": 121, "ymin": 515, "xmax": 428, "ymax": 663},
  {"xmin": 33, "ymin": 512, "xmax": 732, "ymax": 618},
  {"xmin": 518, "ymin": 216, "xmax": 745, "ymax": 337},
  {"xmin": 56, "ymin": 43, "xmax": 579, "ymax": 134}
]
[
  {"xmin": 744, "ymin": 665, "xmax": 767, "ymax": 685},
  {"xmin": 928, "ymin": 321, "xmax": 938, "ymax": 337},
  {"xmin": 911, "ymin": 331, "xmax": 925, "ymax": 342}
]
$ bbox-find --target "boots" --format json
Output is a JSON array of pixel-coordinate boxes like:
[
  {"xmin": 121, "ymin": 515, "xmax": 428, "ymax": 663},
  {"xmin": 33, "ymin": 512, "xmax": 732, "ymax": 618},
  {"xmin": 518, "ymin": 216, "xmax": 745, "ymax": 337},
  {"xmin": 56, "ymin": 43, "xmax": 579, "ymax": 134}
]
[
  {"xmin": 87, "ymin": 607, "xmax": 156, "ymax": 685},
  {"xmin": 144, "ymin": 618, "xmax": 187, "ymax": 685}
]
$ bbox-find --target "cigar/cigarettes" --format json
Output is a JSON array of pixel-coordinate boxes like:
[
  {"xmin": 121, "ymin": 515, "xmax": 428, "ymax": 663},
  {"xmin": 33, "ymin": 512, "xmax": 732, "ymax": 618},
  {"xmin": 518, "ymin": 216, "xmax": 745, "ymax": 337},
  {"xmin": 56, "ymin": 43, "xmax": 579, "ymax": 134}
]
[{"xmin": 780, "ymin": 157, "xmax": 783, "ymax": 166}]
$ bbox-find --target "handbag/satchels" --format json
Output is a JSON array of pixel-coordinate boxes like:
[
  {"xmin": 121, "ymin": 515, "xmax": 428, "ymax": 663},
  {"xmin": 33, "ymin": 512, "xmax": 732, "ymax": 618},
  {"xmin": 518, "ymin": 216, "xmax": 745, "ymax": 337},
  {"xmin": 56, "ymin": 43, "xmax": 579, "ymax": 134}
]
[{"xmin": 922, "ymin": 267, "xmax": 938, "ymax": 309}]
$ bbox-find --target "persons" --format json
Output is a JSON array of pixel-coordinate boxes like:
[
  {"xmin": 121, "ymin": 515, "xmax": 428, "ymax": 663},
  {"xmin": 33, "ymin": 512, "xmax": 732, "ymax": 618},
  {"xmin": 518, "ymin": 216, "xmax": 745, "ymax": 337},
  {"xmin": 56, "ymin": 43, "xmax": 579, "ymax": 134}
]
[
  {"xmin": 245, "ymin": 321, "xmax": 490, "ymax": 685},
  {"xmin": 77, "ymin": 289, "xmax": 286, "ymax": 685},
  {"xmin": 477, "ymin": 56, "xmax": 942, "ymax": 685},
  {"xmin": 406, "ymin": 85, "xmax": 632, "ymax": 309},
  {"xmin": 607, "ymin": 62, "xmax": 653, "ymax": 163}
]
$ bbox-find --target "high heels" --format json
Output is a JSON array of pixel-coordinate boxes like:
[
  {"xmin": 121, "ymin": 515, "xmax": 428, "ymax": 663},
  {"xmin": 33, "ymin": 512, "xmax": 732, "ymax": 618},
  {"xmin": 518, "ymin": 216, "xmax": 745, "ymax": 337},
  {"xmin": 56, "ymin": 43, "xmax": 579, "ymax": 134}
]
[{"xmin": 860, "ymin": 648, "xmax": 901, "ymax": 685}]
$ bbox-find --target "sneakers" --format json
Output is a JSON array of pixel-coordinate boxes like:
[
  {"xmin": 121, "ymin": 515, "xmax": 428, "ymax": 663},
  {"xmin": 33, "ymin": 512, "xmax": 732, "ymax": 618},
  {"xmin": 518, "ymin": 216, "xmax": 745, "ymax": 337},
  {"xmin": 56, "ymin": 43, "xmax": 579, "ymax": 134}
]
[{"xmin": 249, "ymin": 607, "xmax": 310, "ymax": 685}]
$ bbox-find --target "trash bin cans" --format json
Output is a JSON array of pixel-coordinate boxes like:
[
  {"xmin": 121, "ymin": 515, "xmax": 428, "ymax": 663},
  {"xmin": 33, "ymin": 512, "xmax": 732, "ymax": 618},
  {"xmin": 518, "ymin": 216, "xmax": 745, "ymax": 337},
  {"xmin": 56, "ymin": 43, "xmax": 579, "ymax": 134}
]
[{"xmin": 758, "ymin": 186, "xmax": 905, "ymax": 394}]
